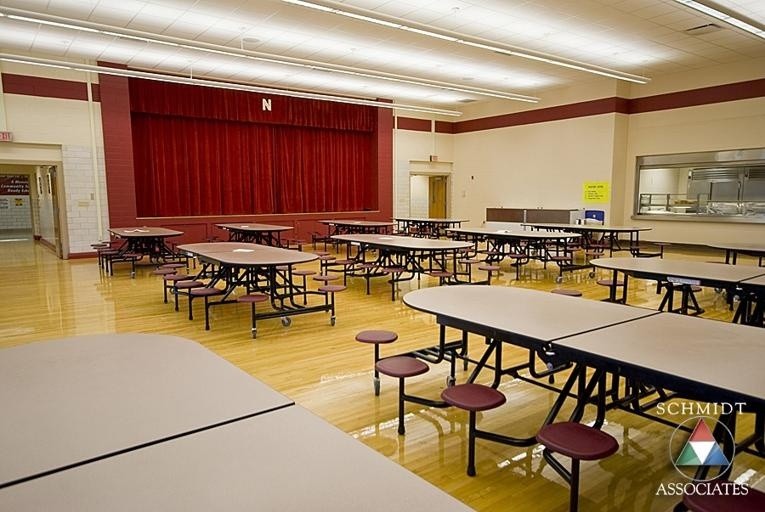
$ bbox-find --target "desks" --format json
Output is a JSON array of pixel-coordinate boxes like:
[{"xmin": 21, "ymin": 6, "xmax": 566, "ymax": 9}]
[
  {"xmin": 217, "ymin": 223, "xmax": 294, "ymax": 247},
  {"xmin": 319, "ymin": 220, "xmax": 396, "ymax": 250},
  {"xmin": 396, "ymin": 218, "xmax": 470, "ymax": 240},
  {"xmin": 0, "ymin": 334, "xmax": 477, "ymax": 512}
]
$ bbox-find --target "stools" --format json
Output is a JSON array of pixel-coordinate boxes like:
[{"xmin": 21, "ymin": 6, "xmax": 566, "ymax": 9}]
[{"xmin": 89, "ymin": 226, "xmax": 765, "ymax": 512}]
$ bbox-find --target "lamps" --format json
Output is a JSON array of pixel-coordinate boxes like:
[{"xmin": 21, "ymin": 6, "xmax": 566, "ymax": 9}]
[{"xmin": 0, "ymin": 0, "xmax": 765, "ymax": 117}]
